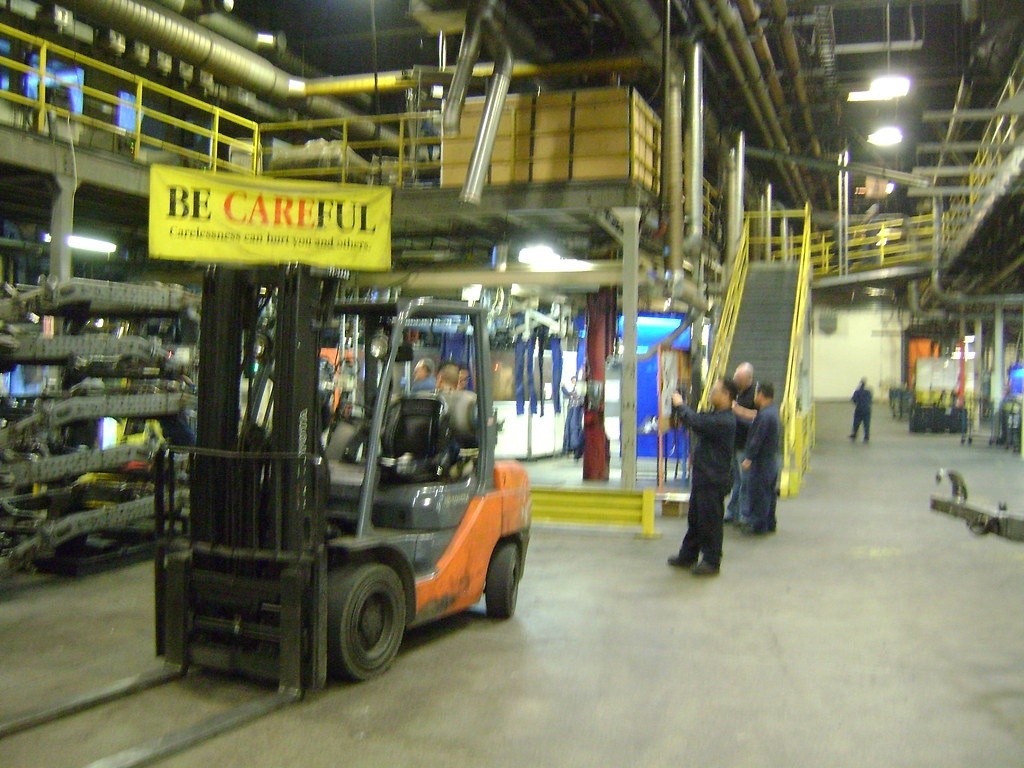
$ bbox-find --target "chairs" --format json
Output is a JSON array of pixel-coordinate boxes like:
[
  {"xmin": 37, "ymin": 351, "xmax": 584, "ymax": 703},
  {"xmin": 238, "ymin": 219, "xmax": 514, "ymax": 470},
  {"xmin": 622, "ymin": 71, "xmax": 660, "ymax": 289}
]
[{"xmin": 341, "ymin": 393, "xmax": 451, "ymax": 488}]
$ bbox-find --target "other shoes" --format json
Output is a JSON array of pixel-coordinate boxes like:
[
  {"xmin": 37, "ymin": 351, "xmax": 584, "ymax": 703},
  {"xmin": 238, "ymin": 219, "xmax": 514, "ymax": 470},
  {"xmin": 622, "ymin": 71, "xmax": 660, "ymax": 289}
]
[
  {"xmin": 668, "ymin": 554, "xmax": 691, "ymax": 568},
  {"xmin": 695, "ymin": 561, "xmax": 719, "ymax": 575}
]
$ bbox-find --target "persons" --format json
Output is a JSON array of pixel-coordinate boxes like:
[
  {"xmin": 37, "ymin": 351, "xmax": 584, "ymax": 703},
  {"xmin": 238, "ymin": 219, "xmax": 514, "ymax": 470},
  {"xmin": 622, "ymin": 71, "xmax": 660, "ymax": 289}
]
[
  {"xmin": 848, "ymin": 378, "xmax": 872, "ymax": 445},
  {"xmin": 855, "ymin": 175, "xmax": 886, "ymax": 264},
  {"xmin": 667, "ymin": 376, "xmax": 735, "ymax": 577},
  {"xmin": 411, "ymin": 356, "xmax": 470, "ymax": 392},
  {"xmin": 741, "ymin": 380, "xmax": 781, "ymax": 536},
  {"xmin": 724, "ymin": 361, "xmax": 760, "ymax": 528},
  {"xmin": 1003, "ymin": 395, "xmax": 1022, "ymax": 451}
]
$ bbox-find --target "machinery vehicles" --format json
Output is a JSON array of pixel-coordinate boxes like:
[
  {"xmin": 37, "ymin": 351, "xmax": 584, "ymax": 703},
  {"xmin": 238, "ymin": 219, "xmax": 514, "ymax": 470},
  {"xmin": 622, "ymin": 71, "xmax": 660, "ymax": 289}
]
[
  {"xmin": 0, "ymin": 265, "xmax": 532, "ymax": 767},
  {"xmin": 911, "ymin": 359, "xmax": 968, "ymax": 434}
]
